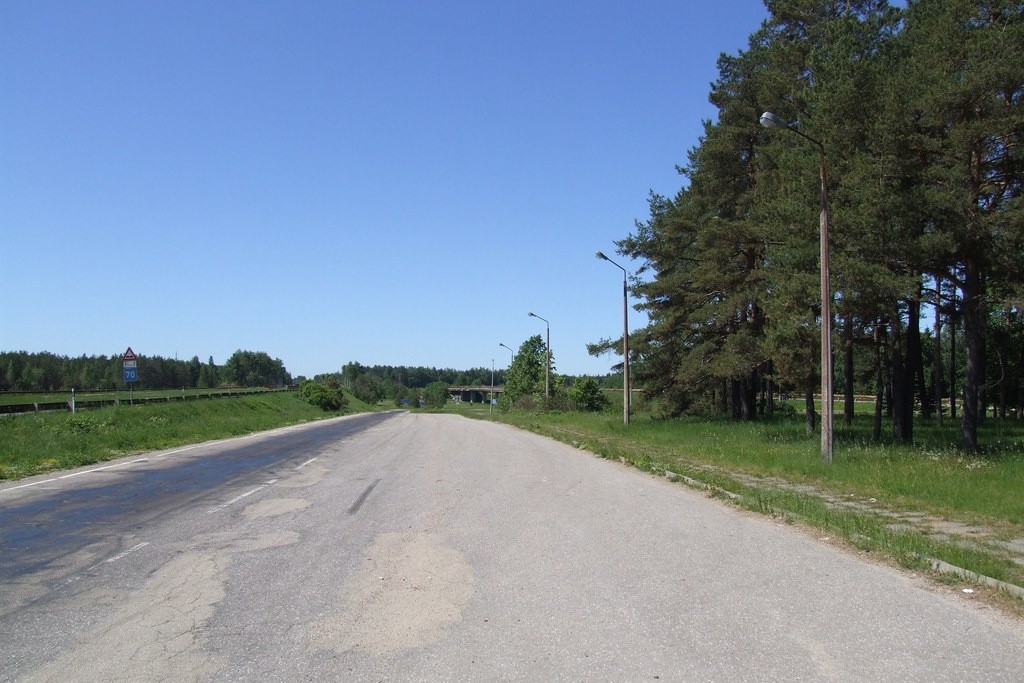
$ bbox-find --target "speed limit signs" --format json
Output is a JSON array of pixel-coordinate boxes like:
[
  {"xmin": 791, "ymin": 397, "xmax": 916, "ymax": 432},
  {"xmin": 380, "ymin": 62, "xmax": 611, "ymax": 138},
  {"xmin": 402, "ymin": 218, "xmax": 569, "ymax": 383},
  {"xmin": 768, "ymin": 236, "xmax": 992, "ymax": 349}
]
[{"xmin": 123, "ymin": 360, "xmax": 137, "ymax": 382}]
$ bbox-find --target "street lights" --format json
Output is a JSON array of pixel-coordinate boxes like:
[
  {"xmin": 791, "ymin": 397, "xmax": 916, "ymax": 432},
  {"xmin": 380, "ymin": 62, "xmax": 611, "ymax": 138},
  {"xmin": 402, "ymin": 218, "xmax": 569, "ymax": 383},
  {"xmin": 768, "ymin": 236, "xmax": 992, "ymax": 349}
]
[
  {"xmin": 527, "ymin": 311, "xmax": 549, "ymax": 403},
  {"xmin": 593, "ymin": 250, "xmax": 629, "ymax": 425},
  {"xmin": 498, "ymin": 342, "xmax": 513, "ymax": 409},
  {"xmin": 759, "ymin": 111, "xmax": 833, "ymax": 464}
]
